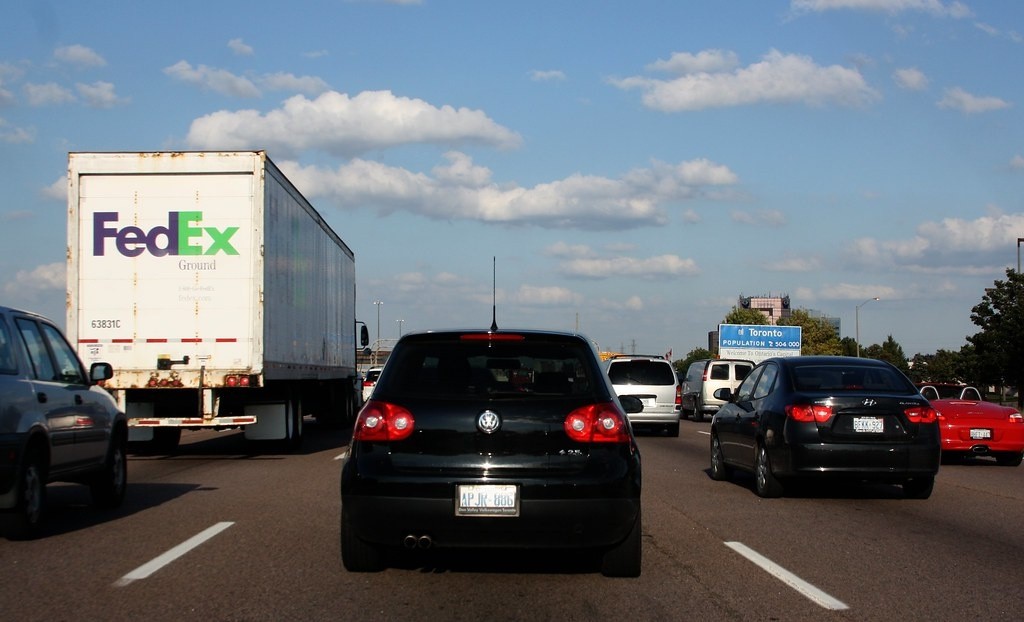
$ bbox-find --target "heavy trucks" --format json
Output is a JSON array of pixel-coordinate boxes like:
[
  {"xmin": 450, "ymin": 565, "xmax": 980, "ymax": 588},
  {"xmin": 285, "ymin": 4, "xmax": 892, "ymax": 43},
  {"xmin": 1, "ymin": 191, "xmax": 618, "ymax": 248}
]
[{"xmin": 65, "ymin": 149, "xmax": 369, "ymax": 453}]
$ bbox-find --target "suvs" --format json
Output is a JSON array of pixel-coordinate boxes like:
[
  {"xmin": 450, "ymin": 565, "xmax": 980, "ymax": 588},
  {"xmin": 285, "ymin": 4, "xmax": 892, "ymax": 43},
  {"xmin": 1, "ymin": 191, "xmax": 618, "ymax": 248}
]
[
  {"xmin": 362, "ymin": 366, "xmax": 382, "ymax": 402},
  {"xmin": 0, "ymin": 307, "xmax": 130, "ymax": 517},
  {"xmin": 602, "ymin": 355, "xmax": 681, "ymax": 437}
]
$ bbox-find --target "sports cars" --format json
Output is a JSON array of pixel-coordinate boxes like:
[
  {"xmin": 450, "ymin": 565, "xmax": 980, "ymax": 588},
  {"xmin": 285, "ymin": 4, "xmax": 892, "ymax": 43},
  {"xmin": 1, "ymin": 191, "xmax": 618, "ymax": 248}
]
[{"xmin": 915, "ymin": 381, "xmax": 1024, "ymax": 469}]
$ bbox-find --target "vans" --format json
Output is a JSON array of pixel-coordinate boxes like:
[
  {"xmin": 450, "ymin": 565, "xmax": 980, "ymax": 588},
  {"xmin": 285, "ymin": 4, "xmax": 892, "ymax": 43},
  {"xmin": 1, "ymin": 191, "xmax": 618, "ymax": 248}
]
[{"xmin": 681, "ymin": 360, "xmax": 755, "ymax": 422}]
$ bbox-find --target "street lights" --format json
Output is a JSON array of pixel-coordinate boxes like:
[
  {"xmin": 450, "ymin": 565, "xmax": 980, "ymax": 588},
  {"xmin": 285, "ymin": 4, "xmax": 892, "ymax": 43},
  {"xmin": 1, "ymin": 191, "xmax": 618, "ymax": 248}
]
[
  {"xmin": 373, "ymin": 300, "xmax": 384, "ymax": 349},
  {"xmin": 855, "ymin": 297, "xmax": 883, "ymax": 356},
  {"xmin": 396, "ymin": 320, "xmax": 404, "ymax": 339}
]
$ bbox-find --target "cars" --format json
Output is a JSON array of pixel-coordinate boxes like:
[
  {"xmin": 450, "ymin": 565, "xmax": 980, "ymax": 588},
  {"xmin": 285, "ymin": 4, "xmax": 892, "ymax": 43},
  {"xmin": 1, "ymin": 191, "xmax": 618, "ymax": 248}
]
[
  {"xmin": 711, "ymin": 355, "xmax": 944, "ymax": 500},
  {"xmin": 340, "ymin": 325, "xmax": 643, "ymax": 579}
]
